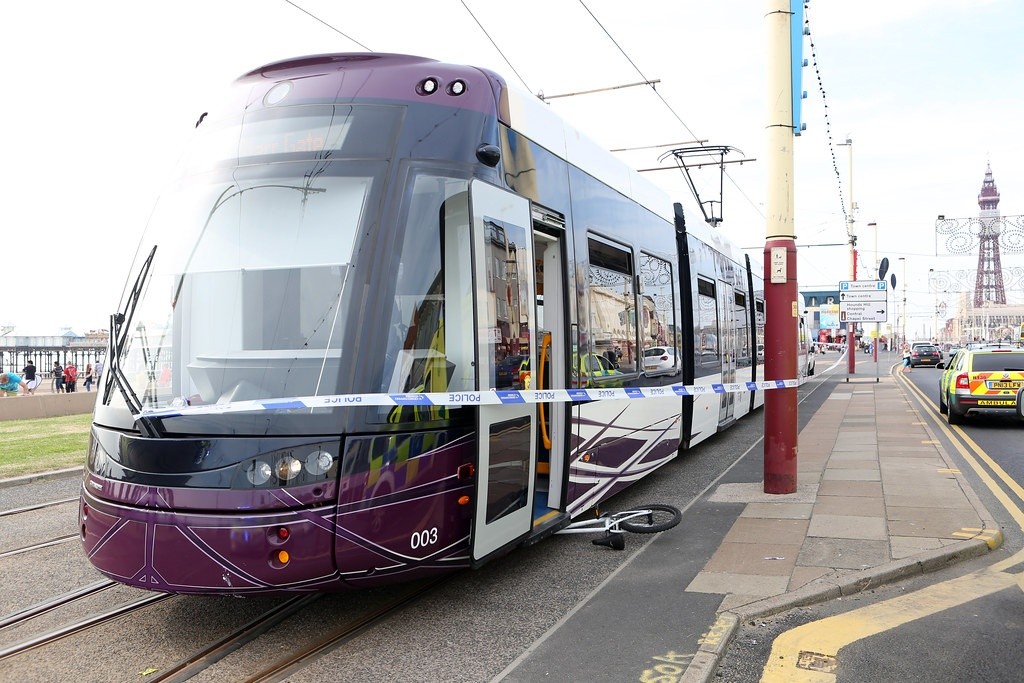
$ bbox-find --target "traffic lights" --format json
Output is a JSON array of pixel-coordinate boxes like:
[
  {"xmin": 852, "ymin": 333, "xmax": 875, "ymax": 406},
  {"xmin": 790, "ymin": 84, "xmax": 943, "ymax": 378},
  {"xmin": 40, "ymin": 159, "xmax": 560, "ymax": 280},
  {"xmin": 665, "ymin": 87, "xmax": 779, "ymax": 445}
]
[{"xmin": 617, "ymin": 311, "xmax": 625, "ymax": 327}]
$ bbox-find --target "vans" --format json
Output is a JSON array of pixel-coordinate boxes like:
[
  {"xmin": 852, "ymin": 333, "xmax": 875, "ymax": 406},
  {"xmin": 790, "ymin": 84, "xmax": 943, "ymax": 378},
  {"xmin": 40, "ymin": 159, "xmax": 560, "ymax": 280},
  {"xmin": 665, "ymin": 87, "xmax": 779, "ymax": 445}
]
[
  {"xmin": 806, "ymin": 330, "xmax": 816, "ymax": 375},
  {"xmin": 743, "ymin": 342, "xmax": 765, "ymax": 359}
]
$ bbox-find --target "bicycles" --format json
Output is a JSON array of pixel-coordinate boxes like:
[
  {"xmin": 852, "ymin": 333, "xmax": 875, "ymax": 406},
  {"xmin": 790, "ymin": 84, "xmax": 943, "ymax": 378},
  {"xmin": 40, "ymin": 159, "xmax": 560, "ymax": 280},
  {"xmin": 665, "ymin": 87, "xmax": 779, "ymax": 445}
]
[{"xmin": 557, "ymin": 502, "xmax": 682, "ymax": 551}]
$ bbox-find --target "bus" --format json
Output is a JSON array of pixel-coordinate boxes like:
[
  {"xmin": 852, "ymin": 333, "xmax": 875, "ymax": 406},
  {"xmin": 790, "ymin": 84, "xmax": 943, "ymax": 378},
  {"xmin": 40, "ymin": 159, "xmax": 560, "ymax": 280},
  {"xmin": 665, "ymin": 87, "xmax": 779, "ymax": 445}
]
[{"xmin": 77, "ymin": 52, "xmax": 819, "ymax": 595}]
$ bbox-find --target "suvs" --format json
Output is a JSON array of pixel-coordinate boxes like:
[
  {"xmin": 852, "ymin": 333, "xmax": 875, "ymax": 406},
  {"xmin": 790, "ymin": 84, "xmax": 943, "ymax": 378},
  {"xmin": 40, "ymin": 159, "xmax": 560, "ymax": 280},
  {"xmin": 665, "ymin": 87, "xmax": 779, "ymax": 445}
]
[{"xmin": 936, "ymin": 348, "xmax": 1024, "ymax": 423}]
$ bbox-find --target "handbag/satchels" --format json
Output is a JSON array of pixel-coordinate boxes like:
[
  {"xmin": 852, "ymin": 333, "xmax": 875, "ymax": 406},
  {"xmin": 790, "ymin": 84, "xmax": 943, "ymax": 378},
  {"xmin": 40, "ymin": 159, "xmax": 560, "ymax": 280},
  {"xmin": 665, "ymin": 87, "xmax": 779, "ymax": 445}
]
[{"xmin": 83, "ymin": 382, "xmax": 86, "ymax": 386}]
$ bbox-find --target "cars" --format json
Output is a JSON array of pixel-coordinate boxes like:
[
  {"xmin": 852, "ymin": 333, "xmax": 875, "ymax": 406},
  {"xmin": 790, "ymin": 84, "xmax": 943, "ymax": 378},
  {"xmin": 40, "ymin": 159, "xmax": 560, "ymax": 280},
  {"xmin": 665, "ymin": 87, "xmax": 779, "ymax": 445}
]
[
  {"xmin": 911, "ymin": 340, "xmax": 930, "ymax": 351},
  {"xmin": 933, "ymin": 341, "xmax": 1014, "ymax": 357},
  {"xmin": 489, "ymin": 347, "xmax": 528, "ymax": 384},
  {"xmin": 356, "ymin": 382, "xmax": 451, "ymax": 500},
  {"xmin": 519, "ymin": 353, "xmax": 626, "ymax": 392},
  {"xmin": 640, "ymin": 341, "xmax": 683, "ymax": 377},
  {"xmin": 910, "ymin": 345, "xmax": 940, "ymax": 369}
]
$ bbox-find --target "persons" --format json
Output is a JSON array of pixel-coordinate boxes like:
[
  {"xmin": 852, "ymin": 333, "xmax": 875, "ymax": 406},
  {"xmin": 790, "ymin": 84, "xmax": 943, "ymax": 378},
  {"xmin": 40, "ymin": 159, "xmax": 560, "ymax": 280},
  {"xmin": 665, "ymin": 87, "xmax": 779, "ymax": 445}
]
[
  {"xmin": 813, "ymin": 336, "xmax": 865, "ymax": 354},
  {"xmin": 898, "ymin": 345, "xmax": 912, "ymax": 373},
  {"xmin": 0, "ymin": 361, "xmax": 103, "ymax": 397},
  {"xmin": 614, "ymin": 340, "xmax": 624, "ymax": 360}
]
